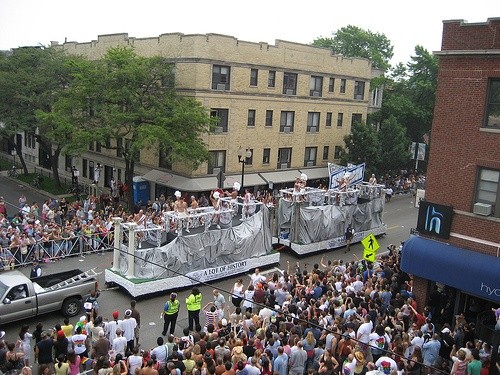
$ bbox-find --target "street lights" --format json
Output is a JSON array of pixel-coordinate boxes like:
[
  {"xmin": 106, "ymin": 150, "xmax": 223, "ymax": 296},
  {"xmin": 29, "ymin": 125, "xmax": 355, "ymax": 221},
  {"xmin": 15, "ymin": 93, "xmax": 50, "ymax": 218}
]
[{"xmin": 237, "ymin": 146, "xmax": 251, "ymax": 197}]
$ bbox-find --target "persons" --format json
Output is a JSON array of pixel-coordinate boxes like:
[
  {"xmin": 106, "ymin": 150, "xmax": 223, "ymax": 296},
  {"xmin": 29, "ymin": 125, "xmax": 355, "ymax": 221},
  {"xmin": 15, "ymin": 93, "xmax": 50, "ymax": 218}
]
[
  {"xmin": 344, "ymin": 224, "xmax": 355, "ymax": 253},
  {"xmin": 30, "ymin": 260, "xmax": 42, "ymax": 278},
  {"xmin": 160, "ymin": 292, "xmax": 180, "ymax": 336},
  {"xmin": 0, "ymin": 245, "xmax": 500, "ymax": 375},
  {"xmin": 71, "ymin": 165, "xmax": 78, "ymax": 183},
  {"xmin": 0, "ymin": 168, "xmax": 427, "ymax": 268},
  {"xmin": 185, "ymin": 287, "xmax": 201, "ymax": 331},
  {"xmin": 94, "ymin": 162, "xmax": 103, "ymax": 184},
  {"xmin": 13, "ymin": 286, "xmax": 26, "ymax": 300}
]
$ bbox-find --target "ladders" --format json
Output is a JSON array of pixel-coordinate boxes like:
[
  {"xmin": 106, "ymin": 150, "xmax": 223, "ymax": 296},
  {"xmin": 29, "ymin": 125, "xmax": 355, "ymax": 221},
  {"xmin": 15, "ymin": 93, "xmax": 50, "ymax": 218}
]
[{"xmin": 45, "ymin": 266, "xmax": 103, "ymax": 292}]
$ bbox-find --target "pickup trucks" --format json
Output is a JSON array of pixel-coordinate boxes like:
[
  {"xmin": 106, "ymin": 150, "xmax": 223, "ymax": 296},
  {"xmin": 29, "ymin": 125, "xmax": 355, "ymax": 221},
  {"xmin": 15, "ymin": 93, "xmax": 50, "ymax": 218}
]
[{"xmin": 0, "ymin": 267, "xmax": 100, "ymax": 330}]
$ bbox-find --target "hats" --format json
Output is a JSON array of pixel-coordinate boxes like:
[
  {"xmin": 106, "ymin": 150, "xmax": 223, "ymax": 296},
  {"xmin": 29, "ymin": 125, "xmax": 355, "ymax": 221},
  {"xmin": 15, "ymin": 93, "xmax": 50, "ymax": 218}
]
[
  {"xmin": 301, "ymin": 173, "xmax": 307, "ymax": 181},
  {"xmin": 116, "ymin": 328, "xmax": 121, "ymax": 335},
  {"xmin": 221, "ymin": 318, "xmax": 227, "ymax": 326},
  {"xmin": 234, "ymin": 346, "xmax": 243, "ymax": 357},
  {"xmin": 441, "ymin": 328, "xmax": 450, "ymax": 333},
  {"xmin": 174, "ymin": 190, "xmax": 181, "ymax": 198},
  {"xmin": 258, "ymin": 283, "xmax": 262, "ymax": 288},
  {"xmin": 354, "ymin": 351, "xmax": 364, "ymax": 362},
  {"xmin": 113, "ymin": 311, "xmax": 119, "ymax": 319},
  {"xmin": 234, "ymin": 182, "xmax": 241, "ymax": 190},
  {"xmin": 124, "ymin": 310, "xmax": 133, "ymax": 317},
  {"xmin": 344, "ymin": 171, "xmax": 350, "ymax": 178},
  {"xmin": 213, "ymin": 191, "xmax": 220, "ymax": 199},
  {"xmin": 98, "ymin": 330, "xmax": 104, "ymax": 337},
  {"xmin": 35, "ymin": 220, "xmax": 40, "ymax": 224}
]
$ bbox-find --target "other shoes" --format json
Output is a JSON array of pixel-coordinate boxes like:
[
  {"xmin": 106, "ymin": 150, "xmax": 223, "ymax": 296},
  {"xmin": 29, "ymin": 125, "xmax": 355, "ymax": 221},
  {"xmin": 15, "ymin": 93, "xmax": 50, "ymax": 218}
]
[
  {"xmin": 216, "ymin": 224, "xmax": 221, "ymax": 229},
  {"xmin": 138, "ymin": 242, "xmax": 141, "ymax": 248},
  {"xmin": 175, "ymin": 229, "xmax": 178, "ymax": 234},
  {"xmin": 185, "ymin": 229, "xmax": 190, "ymax": 233},
  {"xmin": 209, "ymin": 222, "xmax": 212, "ymax": 228}
]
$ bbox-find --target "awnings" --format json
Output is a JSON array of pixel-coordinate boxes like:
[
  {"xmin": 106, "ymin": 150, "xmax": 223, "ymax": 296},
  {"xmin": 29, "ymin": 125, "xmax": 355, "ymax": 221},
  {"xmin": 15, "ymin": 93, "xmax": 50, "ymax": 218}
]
[{"xmin": 400, "ymin": 236, "xmax": 500, "ymax": 304}]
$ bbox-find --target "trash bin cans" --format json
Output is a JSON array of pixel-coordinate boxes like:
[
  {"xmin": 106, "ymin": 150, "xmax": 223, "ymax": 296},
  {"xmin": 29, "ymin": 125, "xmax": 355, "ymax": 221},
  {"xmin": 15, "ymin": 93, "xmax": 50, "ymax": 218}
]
[{"xmin": 131, "ymin": 176, "xmax": 150, "ymax": 206}]
